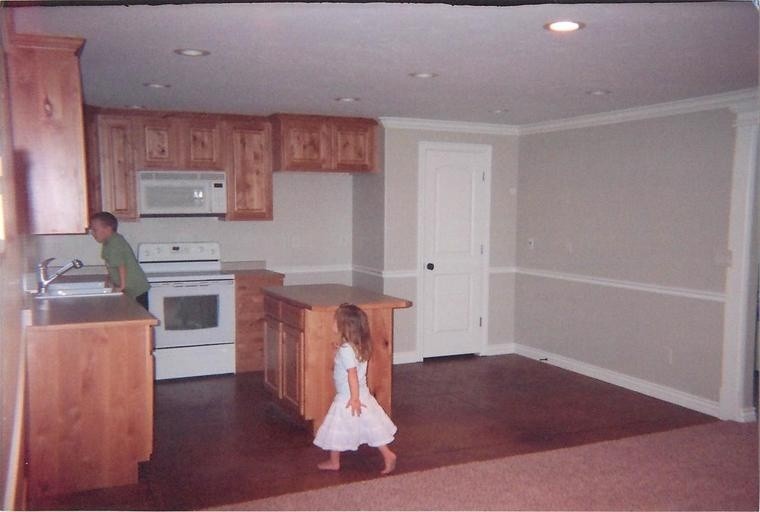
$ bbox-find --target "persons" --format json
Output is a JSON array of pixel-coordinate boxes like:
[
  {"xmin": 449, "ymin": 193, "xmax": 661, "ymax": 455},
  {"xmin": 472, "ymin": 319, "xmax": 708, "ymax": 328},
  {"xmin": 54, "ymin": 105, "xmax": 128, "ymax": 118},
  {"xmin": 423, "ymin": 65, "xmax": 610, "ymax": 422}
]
[
  {"xmin": 90, "ymin": 211, "xmax": 151, "ymax": 311},
  {"xmin": 312, "ymin": 302, "xmax": 398, "ymax": 475}
]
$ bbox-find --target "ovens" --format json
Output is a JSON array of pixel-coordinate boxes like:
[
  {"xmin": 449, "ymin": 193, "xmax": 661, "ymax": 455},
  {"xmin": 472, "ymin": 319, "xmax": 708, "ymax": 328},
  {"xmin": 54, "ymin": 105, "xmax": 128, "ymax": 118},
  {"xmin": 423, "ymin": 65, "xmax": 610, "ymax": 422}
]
[{"xmin": 136, "ymin": 282, "xmax": 236, "ymax": 383}]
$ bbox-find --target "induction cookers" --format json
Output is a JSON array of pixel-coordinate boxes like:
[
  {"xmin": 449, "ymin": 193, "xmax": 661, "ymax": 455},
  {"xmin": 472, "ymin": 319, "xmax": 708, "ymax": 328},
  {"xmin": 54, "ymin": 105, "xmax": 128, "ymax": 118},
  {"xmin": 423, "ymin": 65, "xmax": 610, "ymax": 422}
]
[{"xmin": 130, "ymin": 242, "xmax": 235, "ymax": 281}]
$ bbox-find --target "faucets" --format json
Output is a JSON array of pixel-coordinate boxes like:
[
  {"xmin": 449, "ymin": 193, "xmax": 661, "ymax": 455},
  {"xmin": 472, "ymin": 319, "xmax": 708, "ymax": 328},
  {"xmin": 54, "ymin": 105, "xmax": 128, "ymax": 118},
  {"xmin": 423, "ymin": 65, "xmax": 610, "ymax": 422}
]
[{"xmin": 39, "ymin": 256, "xmax": 84, "ymax": 291}]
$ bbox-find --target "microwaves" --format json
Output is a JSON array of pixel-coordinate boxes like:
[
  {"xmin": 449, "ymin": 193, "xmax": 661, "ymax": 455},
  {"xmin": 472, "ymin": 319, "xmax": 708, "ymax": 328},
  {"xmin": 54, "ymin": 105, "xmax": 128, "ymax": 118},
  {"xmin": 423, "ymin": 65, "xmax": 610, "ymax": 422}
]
[{"xmin": 134, "ymin": 171, "xmax": 229, "ymax": 217}]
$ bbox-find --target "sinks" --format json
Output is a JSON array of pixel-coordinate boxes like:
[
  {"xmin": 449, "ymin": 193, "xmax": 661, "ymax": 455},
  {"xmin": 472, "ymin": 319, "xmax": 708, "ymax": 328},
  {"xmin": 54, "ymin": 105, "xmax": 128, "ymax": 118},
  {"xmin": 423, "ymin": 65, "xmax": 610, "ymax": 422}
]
[
  {"xmin": 48, "ymin": 281, "xmax": 113, "ymax": 291},
  {"xmin": 34, "ymin": 288, "xmax": 126, "ymax": 299}
]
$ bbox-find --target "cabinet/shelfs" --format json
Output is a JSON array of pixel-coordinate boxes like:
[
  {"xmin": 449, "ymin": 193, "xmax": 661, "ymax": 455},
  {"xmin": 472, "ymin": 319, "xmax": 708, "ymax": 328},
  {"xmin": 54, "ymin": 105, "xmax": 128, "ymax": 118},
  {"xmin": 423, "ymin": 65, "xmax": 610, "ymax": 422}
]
[
  {"xmin": 225, "ymin": 117, "xmax": 272, "ymax": 222},
  {"xmin": 26, "ymin": 297, "xmax": 160, "ymax": 495},
  {"xmin": 133, "ymin": 113, "xmax": 224, "ymax": 172},
  {"xmin": 226, "ymin": 270, "xmax": 414, "ymax": 446},
  {"xmin": 10, "ymin": 33, "xmax": 89, "ymax": 235},
  {"xmin": 88, "ymin": 109, "xmax": 138, "ymax": 220},
  {"xmin": 274, "ymin": 114, "xmax": 378, "ymax": 175}
]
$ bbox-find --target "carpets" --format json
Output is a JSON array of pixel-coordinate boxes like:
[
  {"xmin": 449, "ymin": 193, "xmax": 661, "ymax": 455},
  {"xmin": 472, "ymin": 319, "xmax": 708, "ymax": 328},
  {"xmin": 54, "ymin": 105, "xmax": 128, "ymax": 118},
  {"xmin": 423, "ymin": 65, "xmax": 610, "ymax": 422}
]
[{"xmin": 24, "ymin": 345, "xmax": 718, "ymax": 511}]
